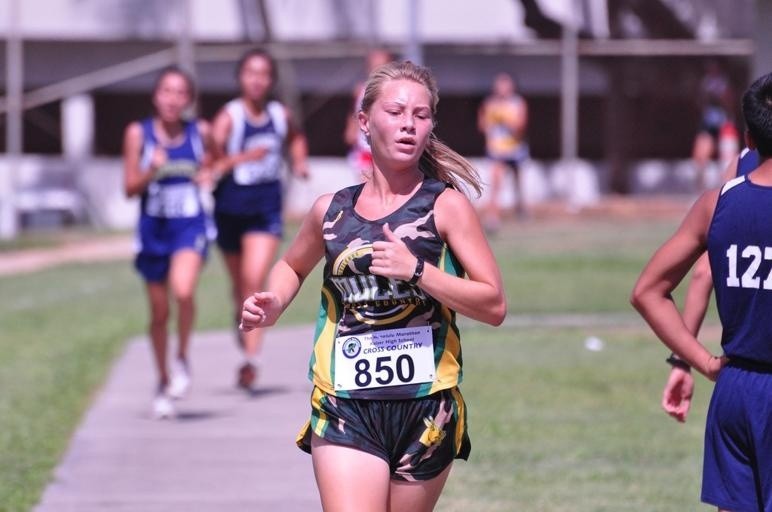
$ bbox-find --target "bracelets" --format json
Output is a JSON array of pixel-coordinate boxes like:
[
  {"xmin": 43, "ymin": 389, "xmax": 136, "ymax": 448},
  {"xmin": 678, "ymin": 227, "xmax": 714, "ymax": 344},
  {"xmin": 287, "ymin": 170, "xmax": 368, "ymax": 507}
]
[
  {"xmin": 407, "ymin": 255, "xmax": 427, "ymax": 291},
  {"xmin": 665, "ymin": 353, "xmax": 694, "ymax": 375}
]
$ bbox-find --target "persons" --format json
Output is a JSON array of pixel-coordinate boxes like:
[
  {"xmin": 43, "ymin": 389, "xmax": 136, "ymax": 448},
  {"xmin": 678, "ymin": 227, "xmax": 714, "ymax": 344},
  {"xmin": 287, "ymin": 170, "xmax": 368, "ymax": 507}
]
[
  {"xmin": 201, "ymin": 48, "xmax": 312, "ymax": 387},
  {"xmin": 659, "ymin": 140, "xmax": 755, "ymax": 424},
  {"xmin": 687, "ymin": 55, "xmax": 736, "ymax": 194},
  {"xmin": 629, "ymin": 71, "xmax": 772, "ymax": 512},
  {"xmin": 345, "ymin": 50, "xmax": 400, "ymax": 177},
  {"xmin": 123, "ymin": 64, "xmax": 215, "ymax": 422},
  {"xmin": 239, "ymin": 60, "xmax": 506, "ymax": 512},
  {"xmin": 475, "ymin": 69, "xmax": 532, "ymax": 232}
]
